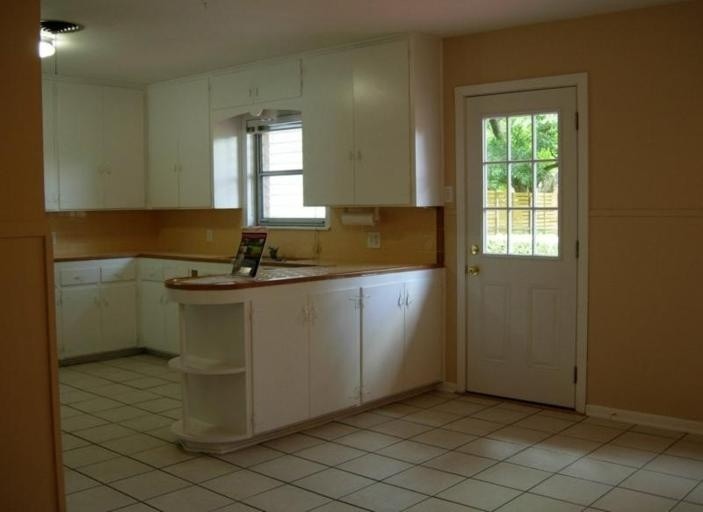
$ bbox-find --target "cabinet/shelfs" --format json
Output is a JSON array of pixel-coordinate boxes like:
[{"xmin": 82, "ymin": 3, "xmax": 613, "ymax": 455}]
[
  {"xmin": 138, "ymin": 260, "xmax": 187, "ymax": 356},
  {"xmin": 57, "ymin": 261, "xmax": 137, "ymax": 360},
  {"xmin": 145, "ymin": 76, "xmax": 240, "ymax": 211},
  {"xmin": 249, "ymin": 274, "xmax": 441, "ymax": 444},
  {"xmin": 301, "ymin": 39, "xmax": 443, "ymax": 208},
  {"xmin": 209, "ymin": 57, "xmax": 301, "ymax": 112},
  {"xmin": 165, "ymin": 289, "xmax": 250, "ymax": 446},
  {"xmin": 56, "ymin": 83, "xmax": 145, "ymax": 212}
]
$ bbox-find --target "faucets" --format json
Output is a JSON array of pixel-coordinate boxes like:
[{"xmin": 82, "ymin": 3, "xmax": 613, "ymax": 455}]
[{"xmin": 267, "ymin": 246, "xmax": 278, "ymax": 259}]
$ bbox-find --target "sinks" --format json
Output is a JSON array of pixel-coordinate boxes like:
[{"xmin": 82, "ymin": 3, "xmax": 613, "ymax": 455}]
[{"xmin": 229, "ymin": 254, "xmax": 313, "ymax": 260}]
